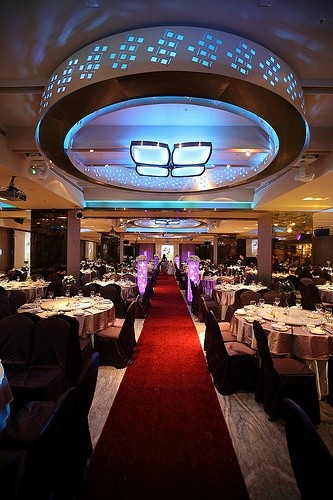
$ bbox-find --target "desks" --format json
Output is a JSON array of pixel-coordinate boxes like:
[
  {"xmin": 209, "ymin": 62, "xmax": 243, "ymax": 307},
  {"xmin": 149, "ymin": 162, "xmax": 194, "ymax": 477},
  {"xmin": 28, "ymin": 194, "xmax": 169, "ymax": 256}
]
[
  {"xmin": 316, "ymin": 284, "xmax": 333, "ymax": 304},
  {"xmin": 0, "ymin": 280, "xmax": 51, "ymax": 302},
  {"xmin": 203, "ymin": 275, "xmax": 235, "ymax": 298},
  {"xmin": 17, "ymin": 296, "xmax": 115, "ymax": 353},
  {"xmin": 85, "ymin": 280, "xmax": 138, "ymax": 304},
  {"xmin": 228, "ymin": 305, "xmax": 333, "ymax": 403},
  {"xmin": 212, "ymin": 283, "xmax": 267, "ymax": 321}
]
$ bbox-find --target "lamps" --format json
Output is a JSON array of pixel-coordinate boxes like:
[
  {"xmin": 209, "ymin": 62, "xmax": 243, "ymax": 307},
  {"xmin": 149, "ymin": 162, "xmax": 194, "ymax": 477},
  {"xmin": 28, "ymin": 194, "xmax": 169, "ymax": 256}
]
[
  {"xmin": 126, "ymin": 215, "xmax": 211, "ymax": 232},
  {"xmin": 118, "ymin": 219, "xmax": 125, "ymax": 229},
  {"xmin": 293, "ymin": 161, "xmax": 315, "ymax": 183},
  {"xmin": 74, "ymin": 206, "xmax": 84, "ymax": 220},
  {"xmin": 27, "ymin": 154, "xmax": 49, "ymax": 181},
  {"xmin": 35, "ymin": 25, "xmax": 311, "ymax": 195}
]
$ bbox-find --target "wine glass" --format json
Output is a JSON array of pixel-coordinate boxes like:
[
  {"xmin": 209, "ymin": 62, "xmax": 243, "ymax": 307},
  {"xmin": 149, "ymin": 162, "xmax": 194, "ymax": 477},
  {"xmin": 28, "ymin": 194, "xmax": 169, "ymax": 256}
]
[
  {"xmin": 244, "ymin": 298, "xmax": 333, "ymax": 333},
  {"xmin": 31, "ymin": 289, "xmax": 104, "ymax": 315}
]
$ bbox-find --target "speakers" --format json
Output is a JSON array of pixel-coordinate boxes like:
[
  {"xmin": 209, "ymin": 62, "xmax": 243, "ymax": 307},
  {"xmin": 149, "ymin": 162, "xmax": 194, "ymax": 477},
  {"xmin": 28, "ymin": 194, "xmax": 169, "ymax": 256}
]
[
  {"xmin": 314, "ymin": 229, "xmax": 330, "ymax": 236},
  {"xmin": 204, "ymin": 241, "xmax": 211, "ymax": 245},
  {"xmin": 123, "ymin": 240, "xmax": 129, "ymax": 244}
]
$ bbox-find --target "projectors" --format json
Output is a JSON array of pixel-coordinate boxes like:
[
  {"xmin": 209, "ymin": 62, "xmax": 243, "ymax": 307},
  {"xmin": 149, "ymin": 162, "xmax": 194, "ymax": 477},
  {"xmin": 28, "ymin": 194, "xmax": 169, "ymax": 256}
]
[{"xmin": 0, "ymin": 189, "xmax": 27, "ymax": 201}]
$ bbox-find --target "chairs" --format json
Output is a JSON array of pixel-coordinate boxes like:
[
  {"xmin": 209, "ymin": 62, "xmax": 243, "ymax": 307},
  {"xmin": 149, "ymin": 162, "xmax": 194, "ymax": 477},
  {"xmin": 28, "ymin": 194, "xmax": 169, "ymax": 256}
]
[
  {"xmin": 173, "ymin": 262, "xmax": 333, "ymax": 500},
  {"xmin": 0, "ymin": 259, "xmax": 161, "ymax": 500}
]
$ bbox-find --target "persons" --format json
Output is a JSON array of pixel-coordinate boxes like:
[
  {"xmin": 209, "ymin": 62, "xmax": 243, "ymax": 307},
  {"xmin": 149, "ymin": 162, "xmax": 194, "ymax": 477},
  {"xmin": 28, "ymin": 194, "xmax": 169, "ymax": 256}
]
[
  {"xmin": 161, "ymin": 254, "xmax": 168, "ymax": 275},
  {"xmin": 167, "ymin": 260, "xmax": 173, "ymax": 275}
]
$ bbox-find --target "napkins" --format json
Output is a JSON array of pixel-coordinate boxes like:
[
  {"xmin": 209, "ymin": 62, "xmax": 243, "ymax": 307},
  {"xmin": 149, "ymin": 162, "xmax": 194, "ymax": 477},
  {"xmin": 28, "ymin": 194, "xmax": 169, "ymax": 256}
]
[{"xmin": 309, "ymin": 327, "xmax": 326, "ymax": 335}]
